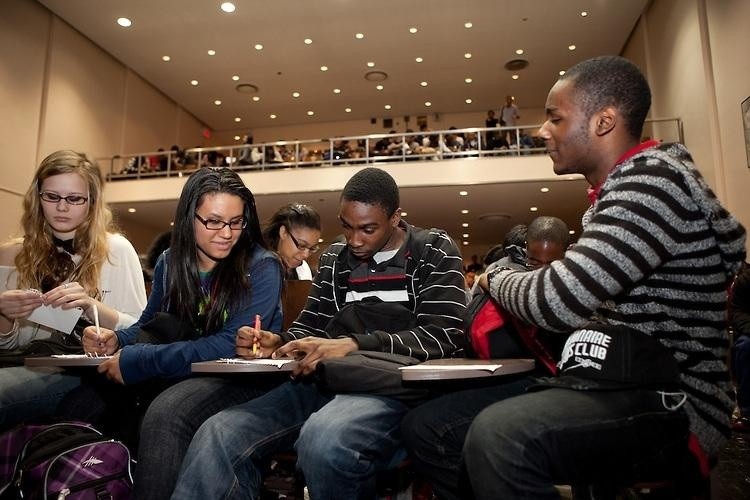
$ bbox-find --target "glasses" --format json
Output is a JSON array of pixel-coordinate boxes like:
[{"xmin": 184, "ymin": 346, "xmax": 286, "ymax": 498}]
[
  {"xmin": 38, "ymin": 191, "xmax": 89, "ymax": 205},
  {"xmin": 192, "ymin": 211, "xmax": 248, "ymax": 231},
  {"xmin": 285, "ymin": 227, "xmax": 320, "ymax": 253}
]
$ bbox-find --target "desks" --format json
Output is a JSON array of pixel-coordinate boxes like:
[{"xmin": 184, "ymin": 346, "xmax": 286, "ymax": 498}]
[
  {"xmin": 187, "ymin": 355, "xmax": 306, "ymax": 378},
  {"xmin": 23, "ymin": 354, "xmax": 113, "ymax": 371},
  {"xmin": 398, "ymin": 347, "xmax": 536, "ymax": 383}
]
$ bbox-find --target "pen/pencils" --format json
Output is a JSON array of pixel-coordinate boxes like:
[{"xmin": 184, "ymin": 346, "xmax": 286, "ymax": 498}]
[
  {"xmin": 252, "ymin": 315, "xmax": 262, "ymax": 356},
  {"xmin": 92, "ymin": 305, "xmax": 101, "ymax": 347}
]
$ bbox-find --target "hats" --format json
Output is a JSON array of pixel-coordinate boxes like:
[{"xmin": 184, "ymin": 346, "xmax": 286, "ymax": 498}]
[{"xmin": 523, "ymin": 324, "xmax": 681, "ymax": 402}]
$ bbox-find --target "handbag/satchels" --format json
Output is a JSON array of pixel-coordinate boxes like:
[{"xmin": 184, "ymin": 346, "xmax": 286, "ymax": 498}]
[
  {"xmin": 500, "ymin": 117, "xmax": 506, "ymax": 126},
  {"xmin": 460, "ymin": 289, "xmax": 562, "ymax": 377}
]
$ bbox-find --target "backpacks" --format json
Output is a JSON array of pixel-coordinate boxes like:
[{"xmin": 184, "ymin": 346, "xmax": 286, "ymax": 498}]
[
  {"xmin": 319, "ymin": 294, "xmax": 422, "ymax": 403},
  {"xmin": 0, "ymin": 418, "xmax": 135, "ymax": 500}
]
